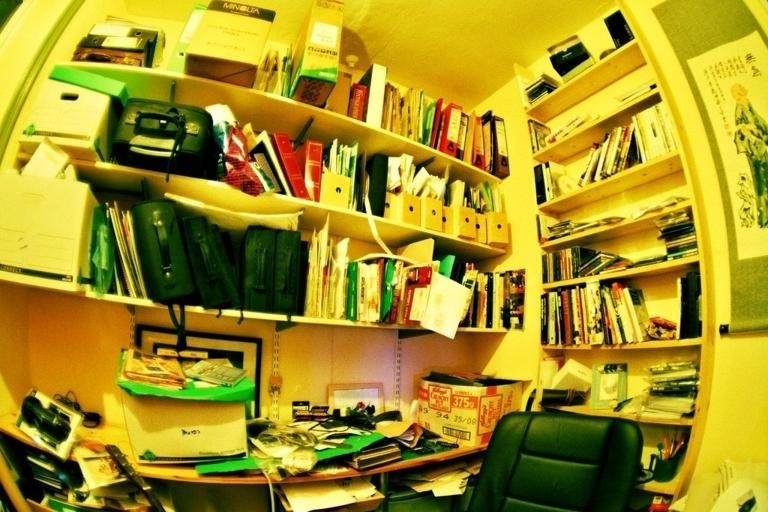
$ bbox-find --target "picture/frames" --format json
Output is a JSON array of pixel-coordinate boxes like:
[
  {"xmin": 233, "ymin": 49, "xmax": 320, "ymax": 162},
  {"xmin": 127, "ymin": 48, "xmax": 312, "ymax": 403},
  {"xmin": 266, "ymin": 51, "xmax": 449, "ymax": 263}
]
[
  {"xmin": 325, "ymin": 380, "xmax": 385, "ymax": 418},
  {"xmin": 129, "ymin": 325, "xmax": 263, "ymax": 420},
  {"xmin": 592, "ymin": 362, "xmax": 630, "ymax": 408}
]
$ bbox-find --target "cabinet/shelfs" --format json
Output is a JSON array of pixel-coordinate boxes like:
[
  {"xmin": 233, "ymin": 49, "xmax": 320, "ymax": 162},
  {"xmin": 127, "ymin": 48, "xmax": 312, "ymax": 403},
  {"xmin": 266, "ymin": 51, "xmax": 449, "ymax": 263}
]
[{"xmin": 2, "ymin": 57, "xmax": 524, "ymax": 333}]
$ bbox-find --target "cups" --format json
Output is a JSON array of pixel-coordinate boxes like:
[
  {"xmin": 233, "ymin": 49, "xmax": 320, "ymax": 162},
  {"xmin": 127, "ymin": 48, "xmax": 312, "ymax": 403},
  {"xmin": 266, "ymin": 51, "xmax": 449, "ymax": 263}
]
[{"xmin": 654, "ymin": 455, "xmax": 681, "ymax": 484}]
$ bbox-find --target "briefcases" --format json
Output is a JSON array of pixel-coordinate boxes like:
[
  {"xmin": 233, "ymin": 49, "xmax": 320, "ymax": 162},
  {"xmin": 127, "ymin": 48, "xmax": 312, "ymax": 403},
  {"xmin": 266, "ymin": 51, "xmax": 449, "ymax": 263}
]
[
  {"xmin": 243, "ymin": 226, "xmax": 276, "ymax": 313},
  {"xmin": 273, "ymin": 229, "xmax": 302, "ymax": 316},
  {"xmin": 131, "ymin": 200, "xmax": 196, "ymax": 302},
  {"xmin": 179, "ymin": 214, "xmax": 241, "ymax": 310}
]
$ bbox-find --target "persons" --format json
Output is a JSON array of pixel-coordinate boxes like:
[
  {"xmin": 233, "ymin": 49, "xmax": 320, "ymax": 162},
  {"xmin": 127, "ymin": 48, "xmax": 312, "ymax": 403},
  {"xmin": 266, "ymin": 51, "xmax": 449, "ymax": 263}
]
[{"xmin": 732, "ymin": 79, "xmax": 768, "ymax": 227}]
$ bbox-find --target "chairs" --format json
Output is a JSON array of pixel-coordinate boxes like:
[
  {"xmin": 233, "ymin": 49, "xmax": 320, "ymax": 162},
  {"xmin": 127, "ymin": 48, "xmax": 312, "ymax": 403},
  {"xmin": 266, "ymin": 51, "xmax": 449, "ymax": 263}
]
[{"xmin": 466, "ymin": 409, "xmax": 644, "ymax": 512}]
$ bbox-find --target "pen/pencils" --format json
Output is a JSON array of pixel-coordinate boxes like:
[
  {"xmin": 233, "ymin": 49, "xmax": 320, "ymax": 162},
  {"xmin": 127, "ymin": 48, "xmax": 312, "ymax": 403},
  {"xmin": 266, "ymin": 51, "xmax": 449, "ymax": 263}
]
[{"xmin": 657, "ymin": 431, "xmax": 685, "ymax": 461}]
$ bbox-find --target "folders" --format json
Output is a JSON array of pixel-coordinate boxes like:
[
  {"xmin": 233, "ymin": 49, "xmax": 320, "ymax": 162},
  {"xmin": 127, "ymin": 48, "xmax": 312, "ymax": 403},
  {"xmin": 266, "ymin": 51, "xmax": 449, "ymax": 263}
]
[
  {"xmin": 472, "ymin": 116, "xmax": 486, "ymax": 170},
  {"xmin": 436, "ymin": 102, "xmax": 463, "ymax": 157},
  {"xmin": 491, "ymin": 115, "xmax": 510, "ymax": 179},
  {"xmin": 72, "ymin": 24, "xmax": 158, "ymax": 68}
]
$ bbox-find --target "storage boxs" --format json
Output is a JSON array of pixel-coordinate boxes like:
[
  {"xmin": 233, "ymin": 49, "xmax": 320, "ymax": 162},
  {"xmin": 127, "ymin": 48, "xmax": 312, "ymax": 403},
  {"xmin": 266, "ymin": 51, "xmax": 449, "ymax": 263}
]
[
  {"xmin": 185, "ymin": 2, "xmax": 347, "ymax": 106},
  {"xmin": 17, "ymin": 65, "xmax": 130, "ymax": 163},
  {"xmin": 414, "ymin": 374, "xmax": 521, "ymax": 445},
  {"xmin": 123, "ymin": 396, "xmax": 248, "ymax": 464},
  {"xmin": 522, "ymin": 40, "xmax": 715, "ymax": 499}
]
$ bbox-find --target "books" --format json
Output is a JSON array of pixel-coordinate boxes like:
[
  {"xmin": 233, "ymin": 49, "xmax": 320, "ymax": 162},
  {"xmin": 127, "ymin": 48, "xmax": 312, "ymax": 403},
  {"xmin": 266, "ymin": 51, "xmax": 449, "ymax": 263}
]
[
  {"xmin": 525, "ymin": 75, "xmax": 677, "ymax": 205},
  {"xmin": 305, "ymin": 137, "xmax": 503, "ymax": 218},
  {"xmin": 306, "ymin": 212, "xmax": 525, "ymax": 330},
  {"xmin": 102, "ymin": 199, "xmax": 149, "ymax": 300},
  {"xmin": 182, "ymin": 358, "xmax": 248, "ymax": 387},
  {"xmin": 344, "ymin": 446, "xmax": 402, "ymax": 470},
  {"xmin": 536, "ymin": 196, "xmax": 702, "ymax": 346},
  {"xmin": 358, "ymin": 437, "xmax": 398, "ymax": 454},
  {"xmin": 347, "ymin": 64, "xmax": 494, "ymax": 173},
  {"xmin": 124, "ymin": 345, "xmax": 187, "ymax": 388}
]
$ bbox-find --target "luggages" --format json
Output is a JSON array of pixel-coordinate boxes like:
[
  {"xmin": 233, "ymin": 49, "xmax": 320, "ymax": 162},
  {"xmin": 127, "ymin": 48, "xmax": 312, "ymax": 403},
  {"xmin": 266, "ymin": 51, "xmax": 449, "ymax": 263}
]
[{"xmin": 112, "ymin": 98, "xmax": 214, "ymax": 175}]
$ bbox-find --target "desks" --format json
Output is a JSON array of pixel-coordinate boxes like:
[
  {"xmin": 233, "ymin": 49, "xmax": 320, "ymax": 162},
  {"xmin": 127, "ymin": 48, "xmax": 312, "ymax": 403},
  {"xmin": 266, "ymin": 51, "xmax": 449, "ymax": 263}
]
[{"xmin": 123, "ymin": 425, "xmax": 485, "ymax": 510}]
